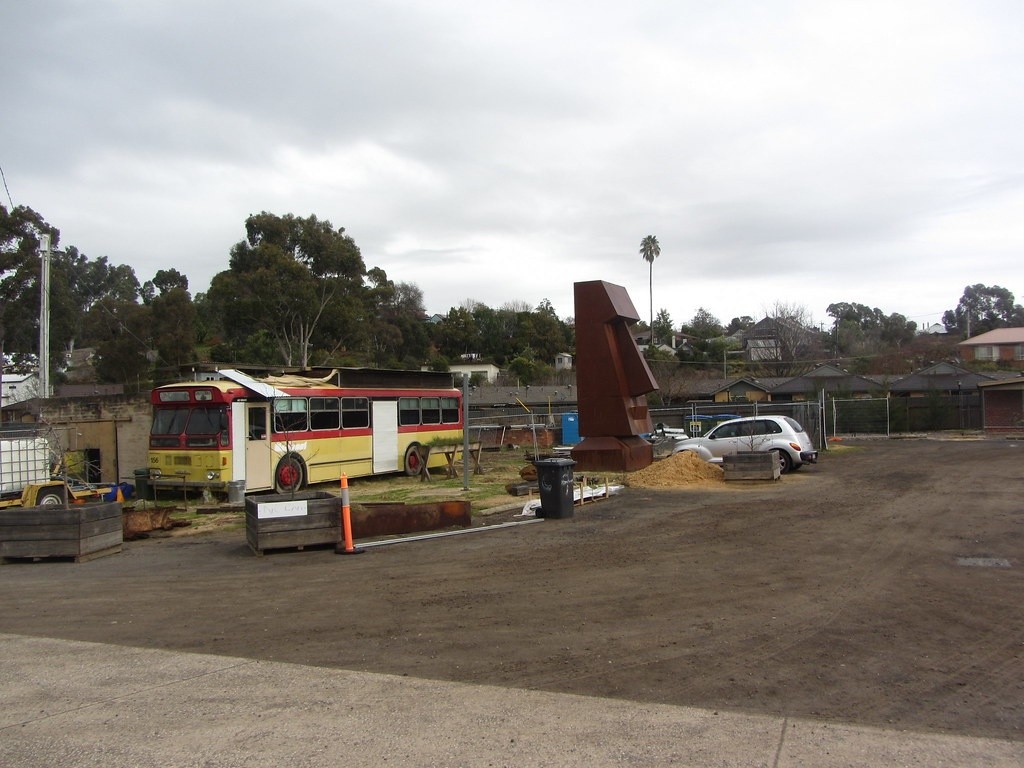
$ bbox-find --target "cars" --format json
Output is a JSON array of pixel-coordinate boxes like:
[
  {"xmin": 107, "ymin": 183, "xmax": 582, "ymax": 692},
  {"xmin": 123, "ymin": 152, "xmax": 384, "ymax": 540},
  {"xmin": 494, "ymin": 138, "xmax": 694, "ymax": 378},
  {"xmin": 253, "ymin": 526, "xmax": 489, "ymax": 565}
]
[{"xmin": 671, "ymin": 415, "xmax": 814, "ymax": 475}]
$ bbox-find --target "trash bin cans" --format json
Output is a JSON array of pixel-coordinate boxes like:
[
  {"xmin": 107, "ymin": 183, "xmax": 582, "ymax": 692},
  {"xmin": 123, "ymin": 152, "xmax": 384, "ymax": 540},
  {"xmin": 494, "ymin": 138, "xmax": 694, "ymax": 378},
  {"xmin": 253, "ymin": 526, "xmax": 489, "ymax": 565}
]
[
  {"xmin": 133, "ymin": 468, "xmax": 155, "ymax": 499},
  {"xmin": 684, "ymin": 415, "xmax": 714, "ymax": 438},
  {"xmin": 711, "ymin": 414, "xmax": 742, "ymax": 432},
  {"xmin": 533, "ymin": 457, "xmax": 578, "ymax": 519}
]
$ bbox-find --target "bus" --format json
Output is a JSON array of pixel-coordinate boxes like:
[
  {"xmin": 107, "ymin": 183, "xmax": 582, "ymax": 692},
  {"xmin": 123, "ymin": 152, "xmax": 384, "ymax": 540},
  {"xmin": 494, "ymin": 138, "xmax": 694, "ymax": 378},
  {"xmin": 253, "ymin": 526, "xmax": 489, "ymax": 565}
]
[{"xmin": 147, "ymin": 375, "xmax": 463, "ymax": 496}]
